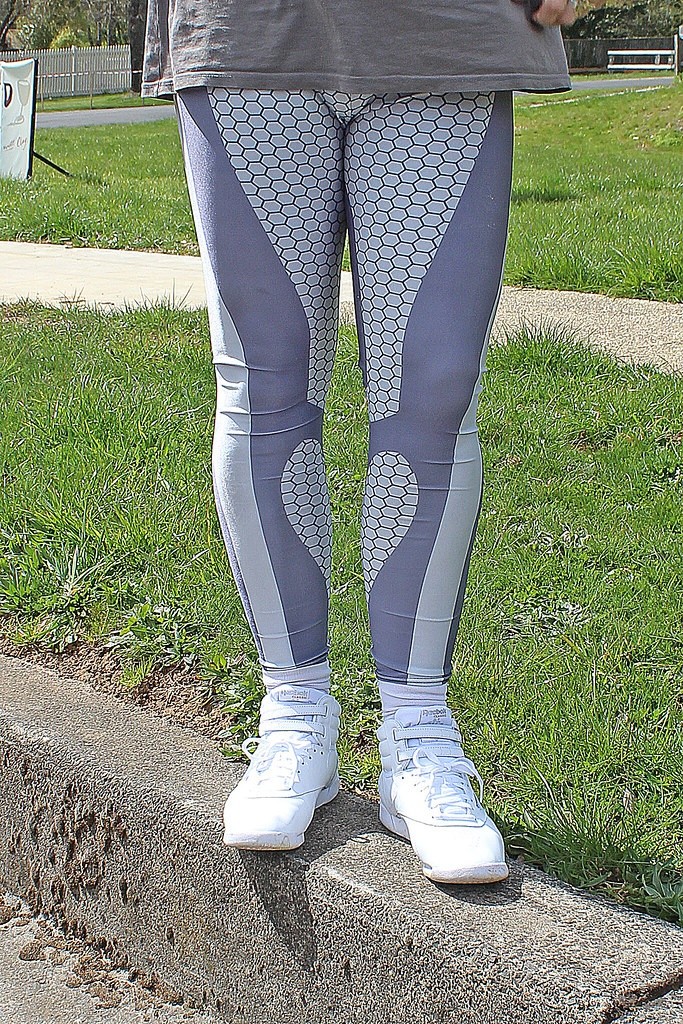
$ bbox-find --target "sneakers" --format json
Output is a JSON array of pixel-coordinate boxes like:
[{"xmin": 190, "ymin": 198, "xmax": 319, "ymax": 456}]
[
  {"xmin": 223, "ymin": 682, "xmax": 341, "ymax": 850},
  {"xmin": 375, "ymin": 705, "xmax": 509, "ymax": 882}
]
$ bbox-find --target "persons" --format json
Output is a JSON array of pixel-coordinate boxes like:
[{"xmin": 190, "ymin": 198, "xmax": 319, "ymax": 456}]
[{"xmin": 141, "ymin": 0, "xmax": 576, "ymax": 883}]
